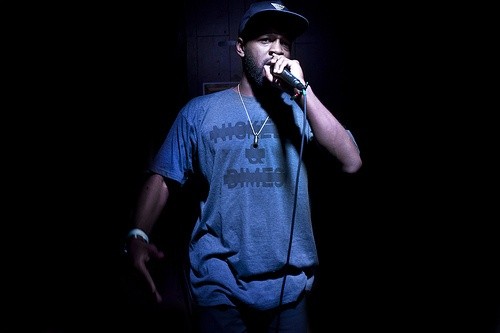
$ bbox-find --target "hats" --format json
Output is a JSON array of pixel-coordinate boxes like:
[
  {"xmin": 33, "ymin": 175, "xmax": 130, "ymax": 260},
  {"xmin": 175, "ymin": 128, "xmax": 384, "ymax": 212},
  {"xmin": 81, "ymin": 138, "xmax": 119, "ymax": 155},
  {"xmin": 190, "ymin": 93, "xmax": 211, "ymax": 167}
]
[{"xmin": 240, "ymin": 0, "xmax": 310, "ymax": 39}]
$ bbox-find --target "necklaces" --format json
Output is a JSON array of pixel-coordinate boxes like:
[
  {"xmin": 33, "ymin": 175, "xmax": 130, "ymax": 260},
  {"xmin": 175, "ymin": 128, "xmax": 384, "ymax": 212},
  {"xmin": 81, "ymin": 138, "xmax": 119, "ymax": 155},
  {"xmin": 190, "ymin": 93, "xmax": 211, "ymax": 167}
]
[{"xmin": 238, "ymin": 84, "xmax": 278, "ymax": 148}]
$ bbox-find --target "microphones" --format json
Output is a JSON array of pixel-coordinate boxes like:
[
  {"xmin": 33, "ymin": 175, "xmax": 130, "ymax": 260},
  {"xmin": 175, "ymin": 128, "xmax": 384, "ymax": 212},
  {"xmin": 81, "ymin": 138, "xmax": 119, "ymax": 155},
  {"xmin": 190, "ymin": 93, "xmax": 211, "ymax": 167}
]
[{"xmin": 266, "ymin": 59, "xmax": 306, "ymax": 92}]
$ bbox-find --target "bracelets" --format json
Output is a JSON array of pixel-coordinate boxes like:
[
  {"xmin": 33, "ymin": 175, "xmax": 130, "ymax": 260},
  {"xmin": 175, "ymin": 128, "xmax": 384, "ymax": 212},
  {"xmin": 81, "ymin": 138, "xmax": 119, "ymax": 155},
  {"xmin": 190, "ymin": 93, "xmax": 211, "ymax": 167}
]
[
  {"xmin": 291, "ymin": 82, "xmax": 309, "ymax": 100},
  {"xmin": 129, "ymin": 228, "xmax": 149, "ymax": 243}
]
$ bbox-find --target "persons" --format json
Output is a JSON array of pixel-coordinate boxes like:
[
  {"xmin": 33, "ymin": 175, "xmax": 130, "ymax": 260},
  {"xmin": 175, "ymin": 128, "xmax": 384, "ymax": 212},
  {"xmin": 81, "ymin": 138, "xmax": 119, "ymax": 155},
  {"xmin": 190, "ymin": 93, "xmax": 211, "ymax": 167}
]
[{"xmin": 123, "ymin": 0, "xmax": 363, "ymax": 333}]
